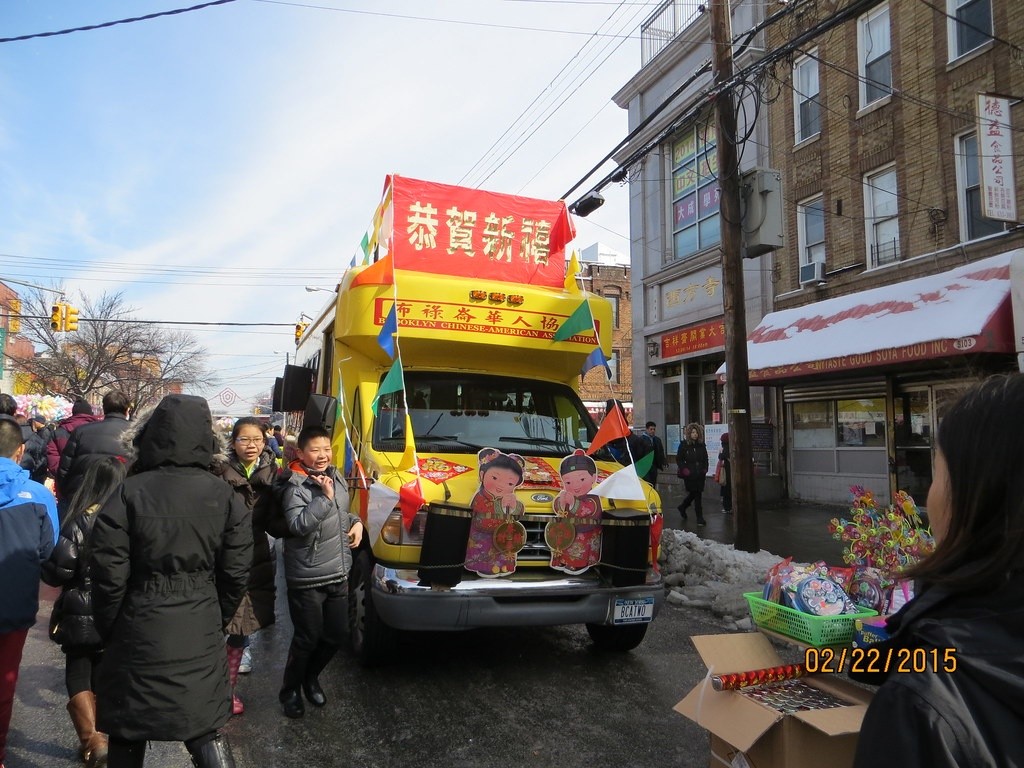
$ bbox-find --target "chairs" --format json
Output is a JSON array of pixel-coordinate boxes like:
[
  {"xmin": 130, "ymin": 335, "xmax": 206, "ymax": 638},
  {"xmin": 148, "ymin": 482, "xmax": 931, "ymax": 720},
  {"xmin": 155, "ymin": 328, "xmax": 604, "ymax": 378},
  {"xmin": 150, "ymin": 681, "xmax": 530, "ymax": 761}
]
[{"xmin": 414, "ymin": 391, "xmax": 457, "ymax": 434}]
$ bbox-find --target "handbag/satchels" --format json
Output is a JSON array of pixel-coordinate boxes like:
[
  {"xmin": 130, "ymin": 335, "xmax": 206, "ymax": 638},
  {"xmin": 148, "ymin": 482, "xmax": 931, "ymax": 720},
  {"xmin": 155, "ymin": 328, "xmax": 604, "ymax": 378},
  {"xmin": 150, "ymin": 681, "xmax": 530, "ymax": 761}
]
[{"xmin": 715, "ymin": 458, "xmax": 726, "ymax": 486}]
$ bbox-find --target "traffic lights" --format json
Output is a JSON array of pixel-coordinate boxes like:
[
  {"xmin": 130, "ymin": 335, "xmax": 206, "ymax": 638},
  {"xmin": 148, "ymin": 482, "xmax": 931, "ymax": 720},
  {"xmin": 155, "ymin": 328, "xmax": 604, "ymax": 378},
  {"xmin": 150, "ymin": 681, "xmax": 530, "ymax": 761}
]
[
  {"xmin": 65, "ymin": 305, "xmax": 79, "ymax": 332},
  {"xmin": 295, "ymin": 322, "xmax": 304, "ymax": 345},
  {"xmin": 50, "ymin": 304, "xmax": 62, "ymax": 331}
]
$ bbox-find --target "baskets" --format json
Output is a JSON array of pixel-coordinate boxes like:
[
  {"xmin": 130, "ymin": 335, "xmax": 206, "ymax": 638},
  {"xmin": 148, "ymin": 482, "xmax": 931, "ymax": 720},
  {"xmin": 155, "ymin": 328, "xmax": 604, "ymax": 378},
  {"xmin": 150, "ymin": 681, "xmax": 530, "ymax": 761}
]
[{"xmin": 745, "ymin": 592, "xmax": 877, "ymax": 646}]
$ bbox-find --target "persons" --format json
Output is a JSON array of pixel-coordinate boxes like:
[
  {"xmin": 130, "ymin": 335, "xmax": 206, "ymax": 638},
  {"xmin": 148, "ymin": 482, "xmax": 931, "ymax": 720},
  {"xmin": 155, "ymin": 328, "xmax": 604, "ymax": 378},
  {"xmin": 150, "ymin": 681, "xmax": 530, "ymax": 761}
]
[
  {"xmin": 281, "ymin": 424, "xmax": 364, "ymax": 719},
  {"xmin": 716, "ymin": 433, "xmax": 732, "ymax": 514},
  {"xmin": 606, "ymin": 399, "xmax": 655, "ymax": 486},
  {"xmin": 217, "ymin": 416, "xmax": 293, "ymax": 714},
  {"xmin": 640, "ymin": 421, "xmax": 669, "ymax": 489},
  {"xmin": 41, "ymin": 499, "xmax": 134, "ymax": 768},
  {"xmin": 853, "ymin": 370, "xmax": 1024, "ymax": 768},
  {"xmin": 0, "ymin": 418, "xmax": 60, "ymax": 768},
  {"xmin": 676, "ymin": 423, "xmax": 708, "ymax": 527},
  {"xmin": 0, "ymin": 388, "xmax": 302, "ymax": 673},
  {"xmin": 88, "ymin": 394, "xmax": 254, "ymax": 768}
]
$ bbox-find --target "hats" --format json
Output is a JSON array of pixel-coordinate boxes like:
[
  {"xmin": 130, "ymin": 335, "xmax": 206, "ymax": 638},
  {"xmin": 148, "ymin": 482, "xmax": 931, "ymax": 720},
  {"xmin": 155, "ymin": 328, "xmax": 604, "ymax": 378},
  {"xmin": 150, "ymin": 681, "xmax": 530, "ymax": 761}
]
[
  {"xmin": 720, "ymin": 433, "xmax": 728, "ymax": 441},
  {"xmin": 31, "ymin": 416, "xmax": 45, "ymax": 424},
  {"xmin": 72, "ymin": 396, "xmax": 92, "ymax": 415}
]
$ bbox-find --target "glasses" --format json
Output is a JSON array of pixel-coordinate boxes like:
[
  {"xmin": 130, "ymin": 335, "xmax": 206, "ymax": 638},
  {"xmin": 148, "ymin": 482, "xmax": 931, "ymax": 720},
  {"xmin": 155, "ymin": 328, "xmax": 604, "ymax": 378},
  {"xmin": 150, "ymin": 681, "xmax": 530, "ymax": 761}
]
[{"xmin": 234, "ymin": 436, "xmax": 264, "ymax": 446}]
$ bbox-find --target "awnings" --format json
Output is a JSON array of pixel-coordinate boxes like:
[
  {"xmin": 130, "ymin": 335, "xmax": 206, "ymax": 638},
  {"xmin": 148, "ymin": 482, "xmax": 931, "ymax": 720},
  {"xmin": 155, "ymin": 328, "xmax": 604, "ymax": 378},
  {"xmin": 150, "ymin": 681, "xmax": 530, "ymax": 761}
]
[{"xmin": 715, "ymin": 247, "xmax": 1013, "ymax": 386}]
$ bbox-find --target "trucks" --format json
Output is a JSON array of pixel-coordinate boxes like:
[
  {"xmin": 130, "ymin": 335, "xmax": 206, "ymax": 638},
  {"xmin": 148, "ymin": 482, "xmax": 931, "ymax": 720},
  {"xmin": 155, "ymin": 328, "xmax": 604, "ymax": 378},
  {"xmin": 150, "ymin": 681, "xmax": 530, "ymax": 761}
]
[{"xmin": 282, "ymin": 264, "xmax": 665, "ymax": 667}]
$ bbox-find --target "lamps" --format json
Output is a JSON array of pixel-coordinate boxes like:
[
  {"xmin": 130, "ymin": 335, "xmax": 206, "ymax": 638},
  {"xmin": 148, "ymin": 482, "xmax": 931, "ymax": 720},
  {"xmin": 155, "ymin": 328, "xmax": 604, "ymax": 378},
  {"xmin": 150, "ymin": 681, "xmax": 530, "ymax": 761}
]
[{"xmin": 647, "ymin": 339, "xmax": 658, "ymax": 358}]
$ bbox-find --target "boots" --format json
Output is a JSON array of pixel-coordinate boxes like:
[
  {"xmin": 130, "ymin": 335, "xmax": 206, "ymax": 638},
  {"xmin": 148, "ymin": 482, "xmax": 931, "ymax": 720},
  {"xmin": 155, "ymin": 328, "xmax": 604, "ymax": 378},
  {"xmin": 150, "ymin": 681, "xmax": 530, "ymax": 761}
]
[
  {"xmin": 227, "ymin": 645, "xmax": 244, "ymax": 714},
  {"xmin": 66, "ymin": 690, "xmax": 110, "ymax": 768},
  {"xmin": 677, "ymin": 497, "xmax": 691, "ymax": 518},
  {"xmin": 191, "ymin": 733, "xmax": 235, "ymax": 768},
  {"xmin": 696, "ymin": 507, "xmax": 706, "ymax": 526}
]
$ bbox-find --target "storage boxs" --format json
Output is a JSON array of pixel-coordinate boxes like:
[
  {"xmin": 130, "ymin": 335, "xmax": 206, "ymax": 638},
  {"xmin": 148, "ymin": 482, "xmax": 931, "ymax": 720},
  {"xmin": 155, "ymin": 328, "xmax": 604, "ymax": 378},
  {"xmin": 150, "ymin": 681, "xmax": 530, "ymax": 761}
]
[
  {"xmin": 673, "ymin": 633, "xmax": 874, "ymax": 768},
  {"xmin": 848, "ymin": 614, "xmax": 896, "ymax": 685}
]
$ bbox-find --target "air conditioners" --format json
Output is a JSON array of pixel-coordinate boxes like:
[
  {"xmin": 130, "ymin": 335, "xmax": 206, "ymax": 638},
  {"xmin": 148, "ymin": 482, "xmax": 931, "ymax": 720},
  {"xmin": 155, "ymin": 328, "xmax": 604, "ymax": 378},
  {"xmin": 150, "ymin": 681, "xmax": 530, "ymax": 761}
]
[{"xmin": 800, "ymin": 262, "xmax": 827, "ymax": 284}]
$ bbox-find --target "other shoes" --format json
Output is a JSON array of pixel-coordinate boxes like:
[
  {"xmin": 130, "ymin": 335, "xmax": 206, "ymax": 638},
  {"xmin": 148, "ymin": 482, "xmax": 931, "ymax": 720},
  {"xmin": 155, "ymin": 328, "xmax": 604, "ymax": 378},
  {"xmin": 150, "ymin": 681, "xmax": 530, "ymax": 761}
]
[
  {"xmin": 239, "ymin": 647, "xmax": 252, "ymax": 672},
  {"xmin": 304, "ymin": 679, "xmax": 326, "ymax": 706},
  {"xmin": 279, "ymin": 688, "xmax": 304, "ymax": 717}
]
